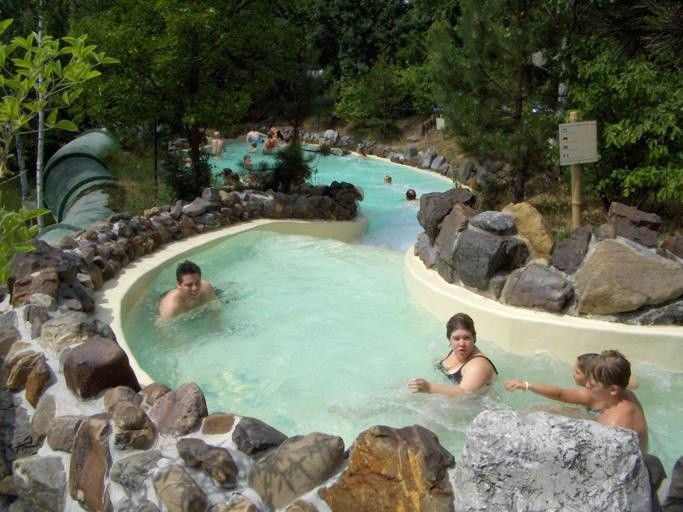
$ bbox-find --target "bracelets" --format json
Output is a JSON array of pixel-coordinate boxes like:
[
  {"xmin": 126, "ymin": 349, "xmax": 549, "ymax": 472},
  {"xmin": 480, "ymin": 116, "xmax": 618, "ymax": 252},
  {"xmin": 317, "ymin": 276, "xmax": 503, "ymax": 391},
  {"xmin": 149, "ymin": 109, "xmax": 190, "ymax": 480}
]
[{"xmin": 524, "ymin": 381, "xmax": 528, "ymax": 392}]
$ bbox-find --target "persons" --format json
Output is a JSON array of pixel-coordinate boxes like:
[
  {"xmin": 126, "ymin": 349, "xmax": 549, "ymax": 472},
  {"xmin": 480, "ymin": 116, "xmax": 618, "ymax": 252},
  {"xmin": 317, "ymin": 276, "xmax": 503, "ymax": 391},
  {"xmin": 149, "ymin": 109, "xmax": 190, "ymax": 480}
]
[
  {"xmin": 159, "ymin": 260, "xmax": 222, "ymax": 340},
  {"xmin": 406, "ymin": 312, "xmax": 499, "ymax": 399},
  {"xmin": 503, "ymin": 352, "xmax": 641, "ymax": 417},
  {"xmin": 383, "ymin": 176, "xmax": 391, "ymax": 186},
  {"xmin": 529, "ymin": 349, "xmax": 649, "ymax": 459},
  {"xmin": 404, "ymin": 189, "xmax": 420, "ymax": 205},
  {"xmin": 208, "ymin": 125, "xmax": 281, "ymax": 192}
]
